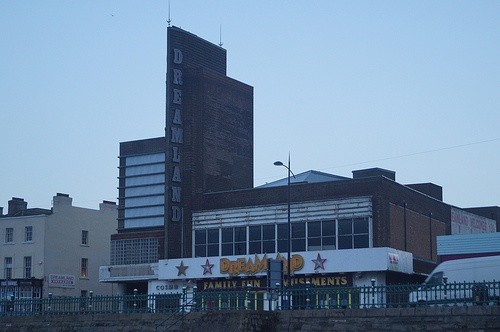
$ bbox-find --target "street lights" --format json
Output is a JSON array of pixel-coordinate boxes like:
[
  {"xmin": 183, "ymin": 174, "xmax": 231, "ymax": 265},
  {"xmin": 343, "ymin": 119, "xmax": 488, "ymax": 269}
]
[{"xmin": 273, "ymin": 152, "xmax": 296, "ymax": 310}]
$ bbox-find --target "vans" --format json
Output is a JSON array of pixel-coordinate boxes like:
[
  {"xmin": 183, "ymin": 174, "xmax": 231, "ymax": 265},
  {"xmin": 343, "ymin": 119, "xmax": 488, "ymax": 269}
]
[{"xmin": 409, "ymin": 255, "xmax": 500, "ymax": 308}]
